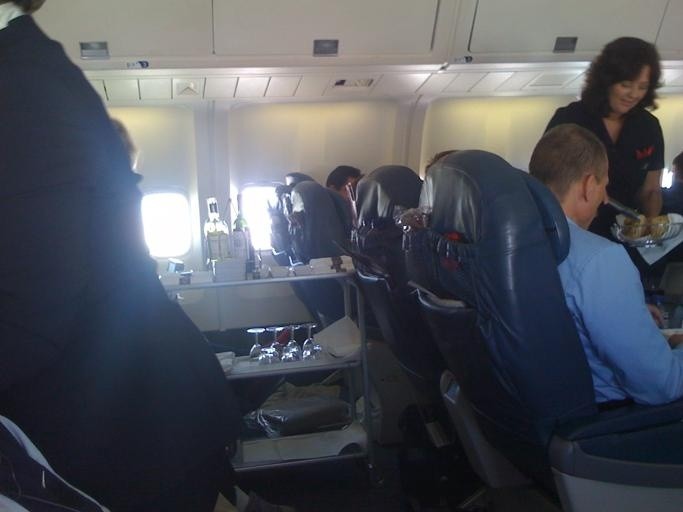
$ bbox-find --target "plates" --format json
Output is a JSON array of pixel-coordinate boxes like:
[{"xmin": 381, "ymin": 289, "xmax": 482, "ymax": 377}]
[{"xmin": 250, "ymin": 266, "xmax": 312, "ymax": 280}]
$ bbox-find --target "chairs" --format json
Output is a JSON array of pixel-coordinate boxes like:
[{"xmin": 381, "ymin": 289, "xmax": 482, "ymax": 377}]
[
  {"xmin": 351, "ymin": 150, "xmax": 683, "ymax": 511},
  {"xmin": 269, "ymin": 173, "xmax": 357, "ymax": 330}
]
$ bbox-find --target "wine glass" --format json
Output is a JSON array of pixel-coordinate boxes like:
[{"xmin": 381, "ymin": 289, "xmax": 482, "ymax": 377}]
[{"xmin": 246, "ymin": 323, "xmax": 322, "ymax": 366}]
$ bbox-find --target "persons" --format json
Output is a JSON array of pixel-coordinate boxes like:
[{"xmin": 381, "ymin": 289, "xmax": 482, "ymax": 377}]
[
  {"xmin": 424, "ymin": 149, "xmax": 461, "ymax": 175},
  {"xmin": 325, "ymin": 165, "xmax": 360, "ymax": 199},
  {"xmin": 528, "ymin": 122, "xmax": 683, "ymax": 405},
  {"xmin": 541, "ymin": 36, "xmax": 666, "ymax": 216},
  {"xmin": 660, "ymin": 152, "xmax": 683, "ymax": 215}
]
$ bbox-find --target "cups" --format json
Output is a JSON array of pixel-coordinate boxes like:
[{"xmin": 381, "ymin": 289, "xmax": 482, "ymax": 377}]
[
  {"xmin": 166, "ymin": 259, "xmax": 184, "ymax": 273},
  {"xmin": 178, "ymin": 273, "xmax": 191, "ymax": 284}
]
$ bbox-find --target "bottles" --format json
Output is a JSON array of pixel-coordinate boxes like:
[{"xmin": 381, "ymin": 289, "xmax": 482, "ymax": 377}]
[
  {"xmin": 232, "ymin": 193, "xmax": 253, "ymax": 273},
  {"xmin": 202, "ymin": 197, "xmax": 230, "ymax": 268}
]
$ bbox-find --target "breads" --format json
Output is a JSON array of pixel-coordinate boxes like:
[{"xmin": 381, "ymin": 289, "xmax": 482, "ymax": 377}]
[{"xmin": 622, "ymin": 215, "xmax": 670, "ymax": 239}]
